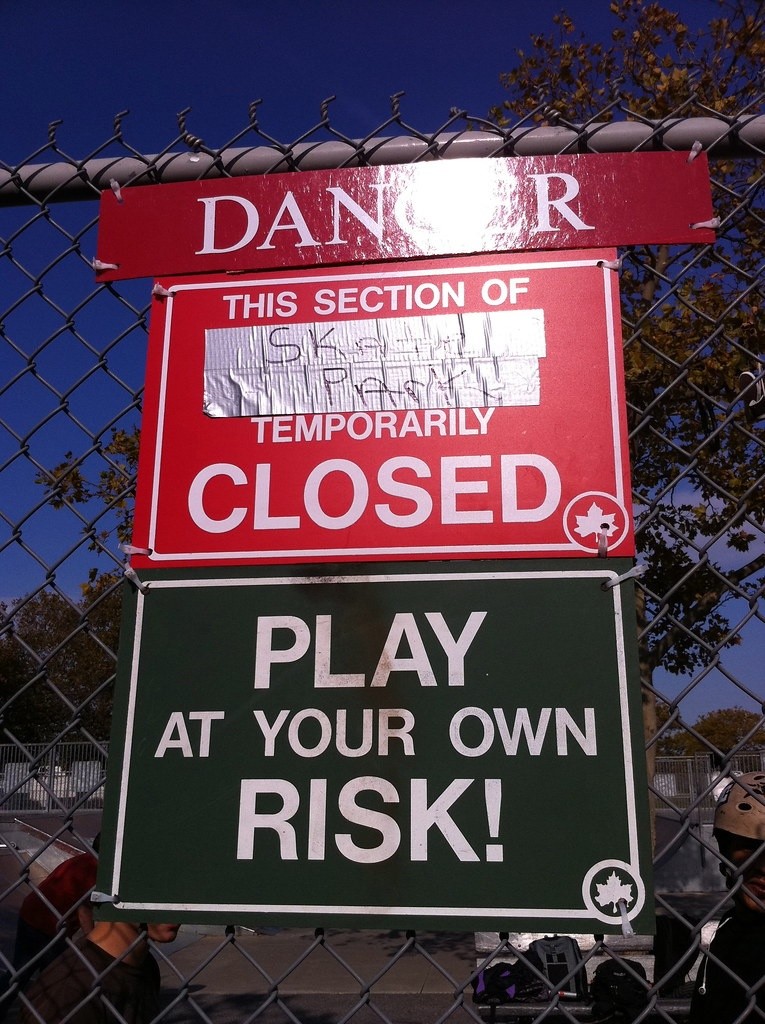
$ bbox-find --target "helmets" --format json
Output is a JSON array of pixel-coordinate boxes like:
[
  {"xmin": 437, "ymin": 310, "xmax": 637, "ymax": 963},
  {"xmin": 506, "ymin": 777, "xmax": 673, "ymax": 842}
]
[{"xmin": 713, "ymin": 772, "xmax": 765, "ymax": 840}]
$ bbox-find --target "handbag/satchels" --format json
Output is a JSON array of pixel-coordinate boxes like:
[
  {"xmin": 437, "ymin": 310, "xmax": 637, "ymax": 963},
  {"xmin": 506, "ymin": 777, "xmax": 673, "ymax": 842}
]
[{"xmin": 514, "ymin": 951, "xmax": 549, "ymax": 1002}]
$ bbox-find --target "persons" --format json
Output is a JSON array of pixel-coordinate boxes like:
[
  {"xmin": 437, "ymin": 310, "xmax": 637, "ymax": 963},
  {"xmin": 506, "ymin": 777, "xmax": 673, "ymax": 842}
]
[
  {"xmin": 689, "ymin": 771, "xmax": 765, "ymax": 1024},
  {"xmin": 0, "ymin": 829, "xmax": 100, "ymax": 1024},
  {"xmin": 17, "ymin": 922, "xmax": 181, "ymax": 1024}
]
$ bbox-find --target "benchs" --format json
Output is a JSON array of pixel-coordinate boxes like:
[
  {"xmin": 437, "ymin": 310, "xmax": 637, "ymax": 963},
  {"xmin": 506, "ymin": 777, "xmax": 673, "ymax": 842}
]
[{"xmin": 478, "ymin": 996, "xmax": 693, "ymax": 1024}]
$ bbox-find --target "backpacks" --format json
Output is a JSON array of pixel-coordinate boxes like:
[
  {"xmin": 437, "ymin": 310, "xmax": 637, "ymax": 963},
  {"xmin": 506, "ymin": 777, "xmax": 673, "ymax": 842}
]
[
  {"xmin": 529, "ymin": 935, "xmax": 588, "ymax": 999},
  {"xmin": 472, "ymin": 962, "xmax": 516, "ymax": 1005},
  {"xmin": 591, "ymin": 958, "xmax": 649, "ymax": 1023}
]
